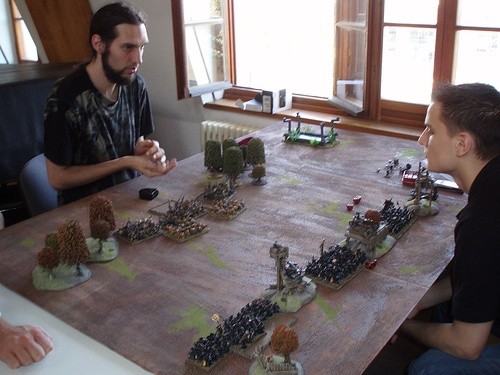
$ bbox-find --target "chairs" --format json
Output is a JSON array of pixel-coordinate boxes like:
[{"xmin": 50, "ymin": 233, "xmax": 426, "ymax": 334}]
[{"xmin": 18, "ymin": 153, "xmax": 57, "ymax": 216}]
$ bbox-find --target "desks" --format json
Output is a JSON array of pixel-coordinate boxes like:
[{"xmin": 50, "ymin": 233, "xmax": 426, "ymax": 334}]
[{"xmin": 0, "ymin": 122, "xmax": 470, "ymax": 375}]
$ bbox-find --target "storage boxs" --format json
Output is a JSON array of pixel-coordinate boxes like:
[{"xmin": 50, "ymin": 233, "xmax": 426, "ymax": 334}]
[{"xmin": 262, "ymin": 87, "xmax": 293, "ymax": 114}]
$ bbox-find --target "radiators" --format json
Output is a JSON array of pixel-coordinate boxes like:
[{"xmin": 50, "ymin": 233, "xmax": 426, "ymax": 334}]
[{"xmin": 201, "ymin": 120, "xmax": 259, "ymax": 152}]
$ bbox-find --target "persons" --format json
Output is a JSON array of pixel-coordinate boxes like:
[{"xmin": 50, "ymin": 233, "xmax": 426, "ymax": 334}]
[
  {"xmin": 40, "ymin": 0, "xmax": 178, "ymax": 208},
  {"xmin": 390, "ymin": 84, "xmax": 500, "ymax": 375}
]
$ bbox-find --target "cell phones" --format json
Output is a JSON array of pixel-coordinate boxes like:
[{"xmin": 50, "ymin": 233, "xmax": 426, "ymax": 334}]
[{"xmin": 434, "ymin": 180, "xmax": 464, "ymax": 194}]
